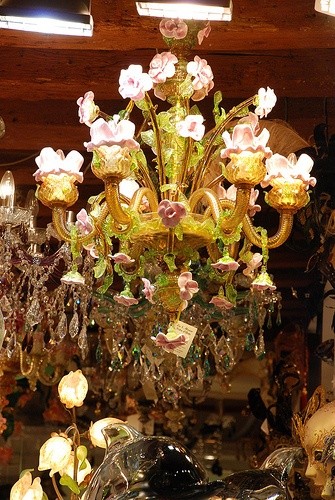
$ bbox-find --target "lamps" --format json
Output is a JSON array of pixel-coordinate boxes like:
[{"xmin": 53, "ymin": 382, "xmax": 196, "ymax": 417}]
[
  {"xmin": 0, "ymin": 166, "xmax": 277, "ymax": 408},
  {"xmin": 10, "ymin": 366, "xmax": 127, "ymax": 500},
  {"xmin": 39, "ymin": 14, "xmax": 316, "ymax": 308}
]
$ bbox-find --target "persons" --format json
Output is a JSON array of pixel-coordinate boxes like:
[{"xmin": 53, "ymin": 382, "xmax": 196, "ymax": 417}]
[
  {"xmin": 319, "ymin": 436, "xmax": 335, "ymax": 500},
  {"xmin": 299, "ymin": 400, "xmax": 335, "ymax": 494}
]
